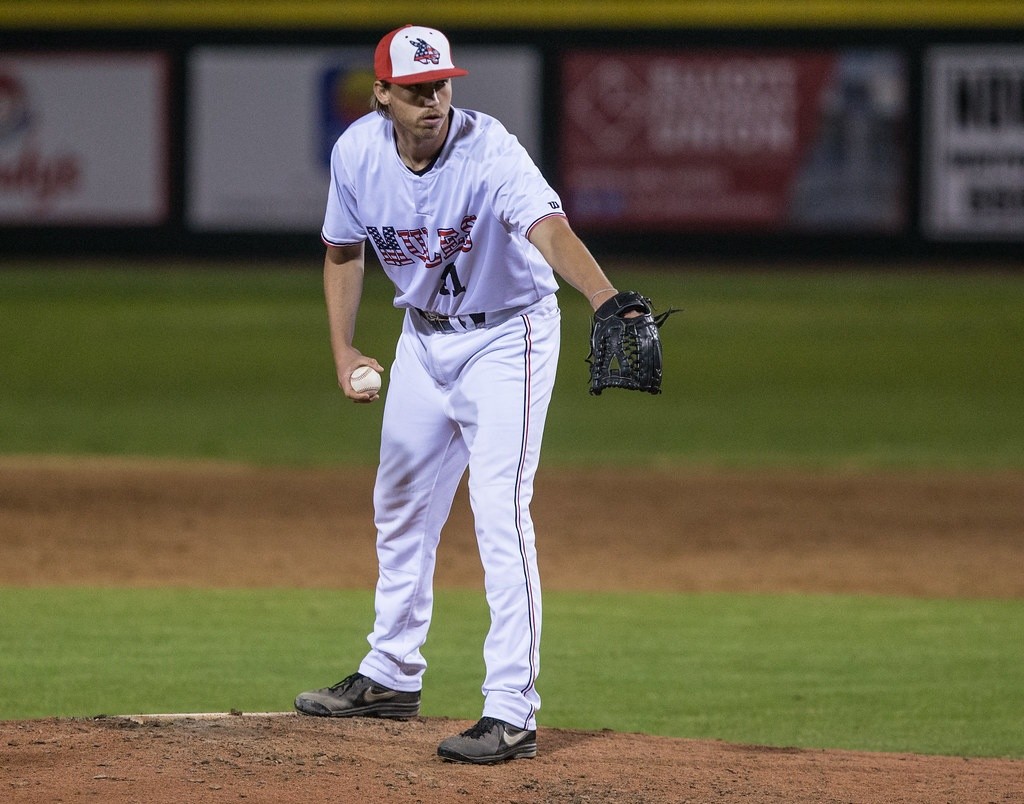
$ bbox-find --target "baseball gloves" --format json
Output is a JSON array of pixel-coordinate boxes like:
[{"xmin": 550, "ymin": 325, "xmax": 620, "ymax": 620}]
[{"xmin": 587, "ymin": 288, "xmax": 663, "ymax": 396}]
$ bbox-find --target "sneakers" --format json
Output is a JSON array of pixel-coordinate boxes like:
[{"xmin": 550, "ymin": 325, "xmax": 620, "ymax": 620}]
[
  {"xmin": 436, "ymin": 717, "xmax": 537, "ymax": 763},
  {"xmin": 294, "ymin": 671, "xmax": 421, "ymax": 720}
]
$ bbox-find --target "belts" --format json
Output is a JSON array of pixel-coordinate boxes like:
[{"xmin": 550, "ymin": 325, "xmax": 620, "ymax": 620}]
[{"xmin": 415, "ymin": 307, "xmax": 485, "ymax": 331}]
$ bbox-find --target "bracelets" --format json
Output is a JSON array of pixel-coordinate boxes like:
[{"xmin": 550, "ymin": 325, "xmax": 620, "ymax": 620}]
[{"xmin": 590, "ymin": 288, "xmax": 618, "ymax": 305}]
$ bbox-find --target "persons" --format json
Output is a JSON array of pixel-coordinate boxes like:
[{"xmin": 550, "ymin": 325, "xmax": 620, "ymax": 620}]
[{"xmin": 295, "ymin": 23, "xmax": 663, "ymax": 766}]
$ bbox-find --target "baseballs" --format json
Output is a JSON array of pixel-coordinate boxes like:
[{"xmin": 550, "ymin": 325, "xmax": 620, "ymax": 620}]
[{"xmin": 350, "ymin": 367, "xmax": 381, "ymax": 397}]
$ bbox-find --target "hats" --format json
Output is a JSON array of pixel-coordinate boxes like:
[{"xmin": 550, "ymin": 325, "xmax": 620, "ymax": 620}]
[{"xmin": 374, "ymin": 24, "xmax": 469, "ymax": 86}]
